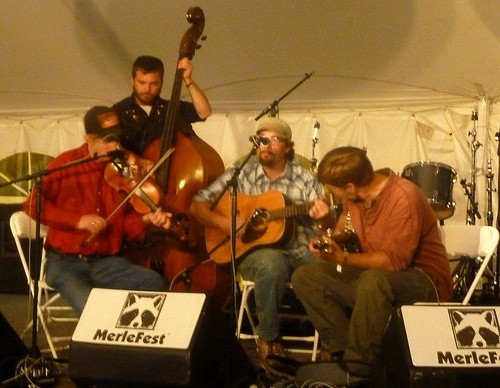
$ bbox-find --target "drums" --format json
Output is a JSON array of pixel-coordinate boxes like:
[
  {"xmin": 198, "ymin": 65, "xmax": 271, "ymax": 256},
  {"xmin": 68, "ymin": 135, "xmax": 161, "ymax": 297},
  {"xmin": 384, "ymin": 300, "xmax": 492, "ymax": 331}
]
[{"xmin": 402, "ymin": 161, "xmax": 457, "ymax": 220}]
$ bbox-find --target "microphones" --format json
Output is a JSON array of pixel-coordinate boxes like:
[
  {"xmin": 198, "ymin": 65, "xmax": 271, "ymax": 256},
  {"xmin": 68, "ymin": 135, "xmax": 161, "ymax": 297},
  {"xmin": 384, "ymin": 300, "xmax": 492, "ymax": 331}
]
[
  {"xmin": 106, "ymin": 149, "xmax": 122, "ymax": 158},
  {"xmin": 249, "ymin": 135, "xmax": 271, "ymax": 145}
]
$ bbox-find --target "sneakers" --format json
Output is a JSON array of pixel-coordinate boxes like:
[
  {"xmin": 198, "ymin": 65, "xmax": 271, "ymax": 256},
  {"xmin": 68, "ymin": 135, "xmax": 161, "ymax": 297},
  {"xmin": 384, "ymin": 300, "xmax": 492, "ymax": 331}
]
[{"xmin": 257, "ymin": 336, "xmax": 289, "ymax": 376}]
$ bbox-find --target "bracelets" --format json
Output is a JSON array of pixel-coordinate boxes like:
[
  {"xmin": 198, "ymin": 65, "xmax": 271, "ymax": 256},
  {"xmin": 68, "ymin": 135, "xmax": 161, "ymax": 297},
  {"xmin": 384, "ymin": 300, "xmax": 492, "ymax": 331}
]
[
  {"xmin": 184, "ymin": 82, "xmax": 192, "ymax": 88},
  {"xmin": 342, "ymin": 252, "xmax": 349, "ymax": 266}
]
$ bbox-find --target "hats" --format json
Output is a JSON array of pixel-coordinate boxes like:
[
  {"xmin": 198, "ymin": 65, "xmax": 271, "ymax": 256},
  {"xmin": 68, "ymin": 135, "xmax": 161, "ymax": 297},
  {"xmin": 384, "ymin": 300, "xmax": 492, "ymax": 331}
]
[
  {"xmin": 255, "ymin": 118, "xmax": 292, "ymax": 141},
  {"xmin": 85, "ymin": 106, "xmax": 125, "ymax": 138}
]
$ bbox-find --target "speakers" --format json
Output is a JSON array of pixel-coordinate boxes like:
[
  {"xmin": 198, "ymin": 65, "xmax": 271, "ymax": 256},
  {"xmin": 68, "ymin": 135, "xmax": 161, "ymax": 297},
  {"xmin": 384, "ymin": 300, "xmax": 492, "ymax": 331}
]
[
  {"xmin": 371, "ymin": 305, "xmax": 500, "ymax": 388},
  {"xmin": 67, "ymin": 286, "xmax": 254, "ymax": 388}
]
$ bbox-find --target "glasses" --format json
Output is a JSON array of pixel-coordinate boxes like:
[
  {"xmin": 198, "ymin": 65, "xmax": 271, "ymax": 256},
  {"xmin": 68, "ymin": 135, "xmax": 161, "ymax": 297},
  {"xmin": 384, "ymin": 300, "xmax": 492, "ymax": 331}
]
[{"xmin": 272, "ymin": 136, "xmax": 287, "ymax": 144}]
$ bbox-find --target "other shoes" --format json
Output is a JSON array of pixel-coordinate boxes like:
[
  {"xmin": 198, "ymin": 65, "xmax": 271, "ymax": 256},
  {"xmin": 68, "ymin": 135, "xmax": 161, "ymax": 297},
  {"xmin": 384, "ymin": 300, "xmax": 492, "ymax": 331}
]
[{"xmin": 265, "ymin": 353, "xmax": 304, "ymax": 377}]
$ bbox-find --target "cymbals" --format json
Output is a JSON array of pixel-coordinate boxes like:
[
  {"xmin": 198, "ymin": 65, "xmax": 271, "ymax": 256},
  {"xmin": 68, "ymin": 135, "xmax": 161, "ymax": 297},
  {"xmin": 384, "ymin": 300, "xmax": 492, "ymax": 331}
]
[{"xmin": 233, "ymin": 150, "xmax": 317, "ymax": 171}]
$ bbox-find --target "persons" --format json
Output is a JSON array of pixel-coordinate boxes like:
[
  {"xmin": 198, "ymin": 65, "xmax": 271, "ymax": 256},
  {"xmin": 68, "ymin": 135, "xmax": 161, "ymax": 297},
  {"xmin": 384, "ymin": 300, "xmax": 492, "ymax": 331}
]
[
  {"xmin": 104, "ymin": 55, "xmax": 213, "ymax": 156},
  {"xmin": 190, "ymin": 119, "xmax": 340, "ymax": 365},
  {"xmin": 22, "ymin": 107, "xmax": 172, "ymax": 320},
  {"xmin": 291, "ymin": 145, "xmax": 453, "ymax": 379}
]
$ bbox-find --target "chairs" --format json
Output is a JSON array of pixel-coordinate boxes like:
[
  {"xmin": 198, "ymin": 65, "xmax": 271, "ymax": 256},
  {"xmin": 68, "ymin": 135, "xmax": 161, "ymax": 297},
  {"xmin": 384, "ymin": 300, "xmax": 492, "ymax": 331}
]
[
  {"xmin": 9, "ymin": 209, "xmax": 79, "ymax": 360},
  {"xmin": 234, "ymin": 280, "xmax": 319, "ymax": 362},
  {"xmin": 437, "ymin": 224, "xmax": 500, "ymax": 305}
]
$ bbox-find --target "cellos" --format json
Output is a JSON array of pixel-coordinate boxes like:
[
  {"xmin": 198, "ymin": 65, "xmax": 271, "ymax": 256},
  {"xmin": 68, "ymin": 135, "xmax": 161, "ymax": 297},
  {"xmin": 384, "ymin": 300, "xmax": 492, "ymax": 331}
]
[{"xmin": 117, "ymin": 7, "xmax": 232, "ymax": 300}]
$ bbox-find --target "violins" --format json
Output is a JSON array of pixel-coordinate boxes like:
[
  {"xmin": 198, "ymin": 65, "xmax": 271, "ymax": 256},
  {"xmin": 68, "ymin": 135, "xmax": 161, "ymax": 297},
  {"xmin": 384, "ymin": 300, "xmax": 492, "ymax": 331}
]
[{"xmin": 104, "ymin": 152, "xmax": 187, "ymax": 241}]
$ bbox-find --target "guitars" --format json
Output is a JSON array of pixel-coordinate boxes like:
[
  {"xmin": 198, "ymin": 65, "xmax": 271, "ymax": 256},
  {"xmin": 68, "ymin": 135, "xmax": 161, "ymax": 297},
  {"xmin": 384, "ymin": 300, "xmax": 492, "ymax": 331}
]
[
  {"xmin": 312, "ymin": 210, "xmax": 361, "ymax": 253},
  {"xmin": 205, "ymin": 189, "xmax": 342, "ymax": 265}
]
